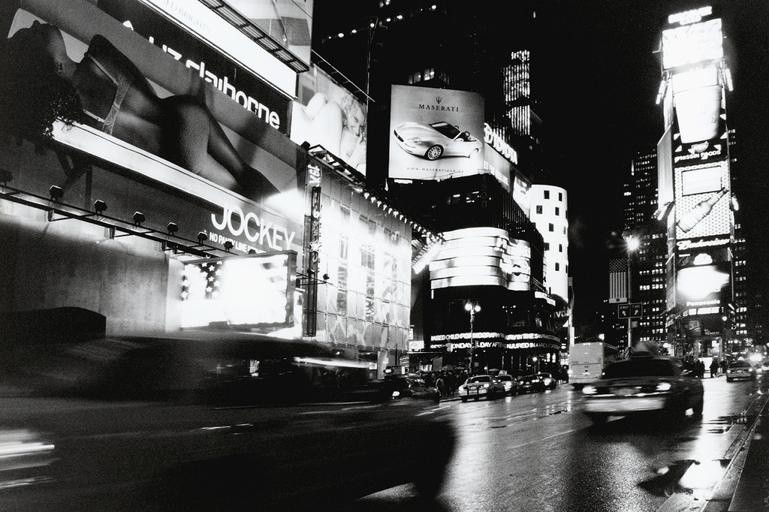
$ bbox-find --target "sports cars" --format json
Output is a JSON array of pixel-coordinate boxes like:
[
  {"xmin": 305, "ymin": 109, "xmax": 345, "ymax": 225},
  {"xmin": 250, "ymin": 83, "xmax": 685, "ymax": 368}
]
[{"xmin": 579, "ymin": 352, "xmax": 703, "ymax": 425}]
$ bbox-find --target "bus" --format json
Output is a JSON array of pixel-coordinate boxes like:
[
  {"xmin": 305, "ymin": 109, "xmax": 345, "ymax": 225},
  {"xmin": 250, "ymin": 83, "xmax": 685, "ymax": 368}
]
[{"xmin": 567, "ymin": 341, "xmax": 621, "ymax": 390}]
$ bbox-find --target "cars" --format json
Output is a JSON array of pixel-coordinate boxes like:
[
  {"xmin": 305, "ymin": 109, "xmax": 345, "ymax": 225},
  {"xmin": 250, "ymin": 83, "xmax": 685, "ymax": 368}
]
[
  {"xmin": 394, "ymin": 120, "xmax": 485, "ymax": 162},
  {"xmin": 725, "ymin": 359, "xmax": 757, "ymax": 382},
  {"xmin": 0, "ymin": 330, "xmax": 460, "ymax": 510},
  {"xmin": 381, "ymin": 370, "xmax": 557, "ymax": 406}
]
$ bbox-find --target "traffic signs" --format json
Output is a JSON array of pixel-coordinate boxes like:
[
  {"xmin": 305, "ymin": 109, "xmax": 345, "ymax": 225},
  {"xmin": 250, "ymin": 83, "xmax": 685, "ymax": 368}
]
[{"xmin": 616, "ymin": 303, "xmax": 643, "ymax": 319}]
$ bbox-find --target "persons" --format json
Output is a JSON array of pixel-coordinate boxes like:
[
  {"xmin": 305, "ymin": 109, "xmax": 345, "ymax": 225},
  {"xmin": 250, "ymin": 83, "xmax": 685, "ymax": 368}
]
[
  {"xmin": 417, "ymin": 368, "xmax": 474, "ymax": 400},
  {"xmin": 680, "ymin": 355, "xmax": 705, "ymax": 378},
  {"xmin": 1, "ymin": 18, "xmax": 303, "ymax": 225},
  {"xmin": 304, "ymin": 87, "xmax": 369, "ymax": 179}
]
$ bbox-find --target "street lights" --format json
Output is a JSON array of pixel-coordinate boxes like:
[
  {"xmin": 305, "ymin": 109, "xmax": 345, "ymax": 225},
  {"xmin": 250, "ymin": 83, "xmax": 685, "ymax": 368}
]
[
  {"xmin": 625, "ymin": 234, "xmax": 641, "ymax": 350},
  {"xmin": 464, "ymin": 298, "xmax": 481, "ymax": 377}
]
